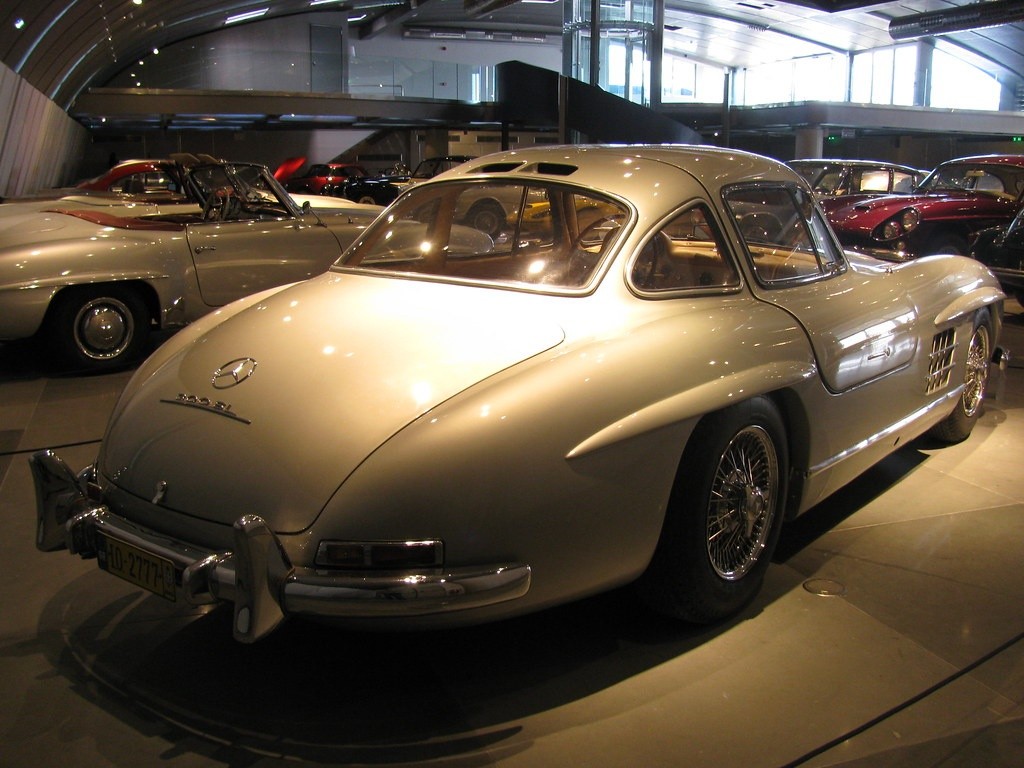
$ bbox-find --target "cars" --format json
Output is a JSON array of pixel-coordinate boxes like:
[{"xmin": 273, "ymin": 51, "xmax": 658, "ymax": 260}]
[
  {"xmin": 504, "ymin": 192, "xmax": 713, "ymax": 245},
  {"xmin": 73, "ymin": 152, "xmax": 235, "ymax": 198},
  {"xmin": 267, "ymin": 150, "xmax": 550, "ymax": 241},
  {"xmin": 693, "ymin": 150, "xmax": 1023, "ymax": 318},
  {"xmin": 1, "ymin": 154, "xmax": 497, "ymax": 372},
  {"xmin": 25, "ymin": 139, "xmax": 1014, "ymax": 647}
]
